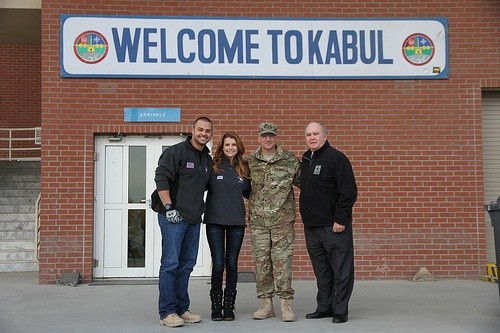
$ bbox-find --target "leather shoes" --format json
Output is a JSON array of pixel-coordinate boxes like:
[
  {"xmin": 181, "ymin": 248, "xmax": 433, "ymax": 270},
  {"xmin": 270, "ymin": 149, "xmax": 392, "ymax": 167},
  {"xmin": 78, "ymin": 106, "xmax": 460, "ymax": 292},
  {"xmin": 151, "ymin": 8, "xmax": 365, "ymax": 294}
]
[
  {"xmin": 305, "ymin": 309, "xmax": 334, "ymax": 319},
  {"xmin": 332, "ymin": 314, "xmax": 348, "ymax": 323}
]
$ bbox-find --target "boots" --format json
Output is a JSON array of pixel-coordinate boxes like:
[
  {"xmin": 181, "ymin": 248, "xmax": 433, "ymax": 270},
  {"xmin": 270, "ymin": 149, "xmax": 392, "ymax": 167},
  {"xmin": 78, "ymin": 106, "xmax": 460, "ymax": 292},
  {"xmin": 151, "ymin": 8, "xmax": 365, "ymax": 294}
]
[
  {"xmin": 209, "ymin": 288, "xmax": 223, "ymax": 320},
  {"xmin": 253, "ymin": 297, "xmax": 275, "ymax": 319},
  {"xmin": 224, "ymin": 288, "xmax": 237, "ymax": 321},
  {"xmin": 279, "ymin": 298, "xmax": 295, "ymax": 322}
]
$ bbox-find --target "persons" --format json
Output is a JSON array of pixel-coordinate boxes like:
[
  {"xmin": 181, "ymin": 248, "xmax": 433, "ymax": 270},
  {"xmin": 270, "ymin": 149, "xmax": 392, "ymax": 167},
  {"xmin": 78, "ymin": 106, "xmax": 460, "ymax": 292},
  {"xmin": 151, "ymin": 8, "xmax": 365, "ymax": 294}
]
[
  {"xmin": 149, "ymin": 116, "xmax": 216, "ymax": 328},
  {"xmin": 201, "ymin": 131, "xmax": 252, "ymax": 321},
  {"xmin": 299, "ymin": 120, "xmax": 358, "ymax": 323},
  {"xmin": 240, "ymin": 121, "xmax": 302, "ymax": 318}
]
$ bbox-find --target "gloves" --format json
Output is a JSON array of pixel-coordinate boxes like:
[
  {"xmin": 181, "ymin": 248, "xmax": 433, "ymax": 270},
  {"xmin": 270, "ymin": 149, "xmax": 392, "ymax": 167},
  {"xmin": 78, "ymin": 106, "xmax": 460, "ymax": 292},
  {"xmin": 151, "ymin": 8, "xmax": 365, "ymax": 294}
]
[{"xmin": 165, "ymin": 208, "xmax": 183, "ymax": 224}]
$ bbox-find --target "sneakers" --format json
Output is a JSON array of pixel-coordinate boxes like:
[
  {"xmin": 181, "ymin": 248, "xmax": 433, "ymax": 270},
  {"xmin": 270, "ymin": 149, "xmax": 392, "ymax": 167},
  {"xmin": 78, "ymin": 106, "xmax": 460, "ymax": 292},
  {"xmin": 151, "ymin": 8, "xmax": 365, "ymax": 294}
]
[
  {"xmin": 181, "ymin": 310, "xmax": 202, "ymax": 323},
  {"xmin": 160, "ymin": 311, "xmax": 185, "ymax": 327}
]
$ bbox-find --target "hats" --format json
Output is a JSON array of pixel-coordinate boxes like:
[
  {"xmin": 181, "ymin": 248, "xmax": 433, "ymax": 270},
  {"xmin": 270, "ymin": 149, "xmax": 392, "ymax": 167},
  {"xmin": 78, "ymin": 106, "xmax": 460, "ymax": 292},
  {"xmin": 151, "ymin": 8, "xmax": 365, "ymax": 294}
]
[{"xmin": 257, "ymin": 121, "xmax": 279, "ymax": 137}]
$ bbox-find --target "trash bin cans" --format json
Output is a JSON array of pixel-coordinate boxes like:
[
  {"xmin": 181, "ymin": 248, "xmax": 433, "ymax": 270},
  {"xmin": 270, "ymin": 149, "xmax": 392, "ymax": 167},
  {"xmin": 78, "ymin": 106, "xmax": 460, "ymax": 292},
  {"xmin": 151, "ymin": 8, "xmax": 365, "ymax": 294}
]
[{"xmin": 484, "ymin": 197, "xmax": 500, "ymax": 319}]
